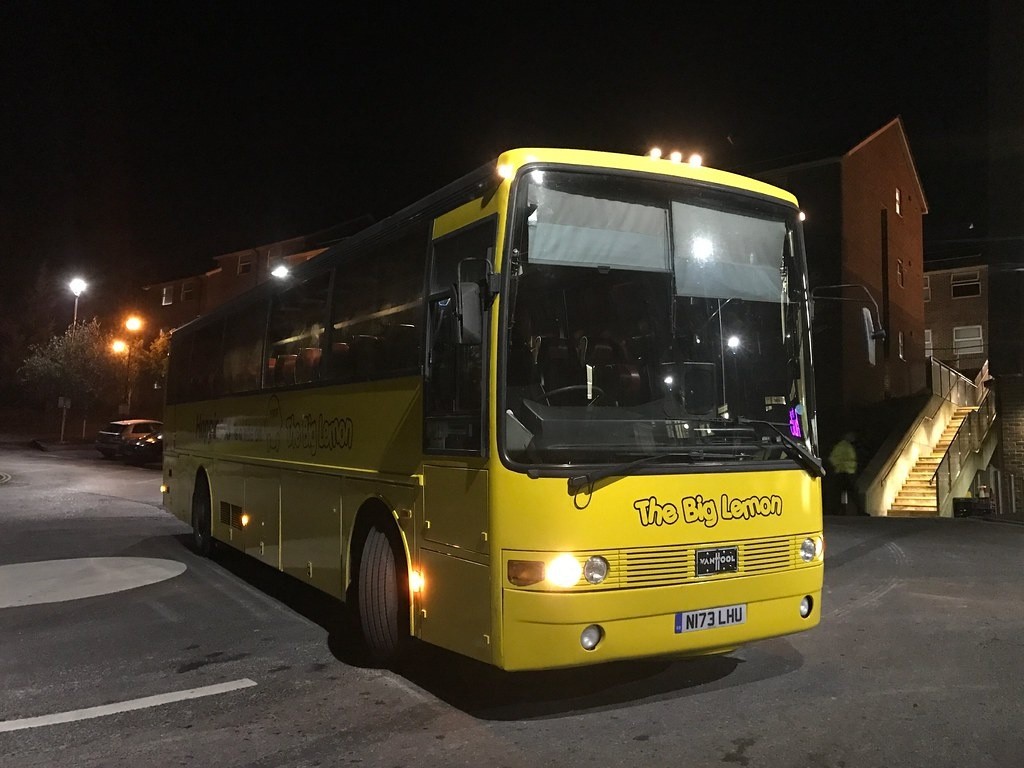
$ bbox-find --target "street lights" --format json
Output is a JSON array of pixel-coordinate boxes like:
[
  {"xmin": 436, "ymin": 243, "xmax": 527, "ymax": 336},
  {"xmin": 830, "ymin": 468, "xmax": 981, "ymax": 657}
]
[
  {"xmin": 123, "ymin": 314, "xmax": 145, "ymax": 417},
  {"xmin": 60, "ymin": 274, "xmax": 91, "ymax": 441}
]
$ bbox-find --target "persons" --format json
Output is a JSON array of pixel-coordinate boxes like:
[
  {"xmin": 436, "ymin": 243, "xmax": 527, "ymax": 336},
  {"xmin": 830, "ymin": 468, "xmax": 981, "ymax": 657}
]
[{"xmin": 829, "ymin": 430, "xmax": 857, "ymax": 515}]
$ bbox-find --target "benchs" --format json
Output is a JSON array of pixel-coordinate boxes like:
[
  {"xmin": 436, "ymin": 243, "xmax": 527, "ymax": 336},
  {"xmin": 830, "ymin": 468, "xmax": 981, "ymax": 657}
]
[{"xmin": 531, "ymin": 335, "xmax": 623, "ymax": 392}]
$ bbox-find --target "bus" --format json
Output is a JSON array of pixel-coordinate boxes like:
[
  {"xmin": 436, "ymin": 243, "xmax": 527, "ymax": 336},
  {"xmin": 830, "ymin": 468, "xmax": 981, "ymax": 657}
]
[{"xmin": 162, "ymin": 147, "xmax": 889, "ymax": 674}]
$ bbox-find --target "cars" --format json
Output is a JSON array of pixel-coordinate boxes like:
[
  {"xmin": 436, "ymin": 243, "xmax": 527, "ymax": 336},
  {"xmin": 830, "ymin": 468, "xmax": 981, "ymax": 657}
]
[{"xmin": 124, "ymin": 427, "xmax": 163, "ymax": 465}]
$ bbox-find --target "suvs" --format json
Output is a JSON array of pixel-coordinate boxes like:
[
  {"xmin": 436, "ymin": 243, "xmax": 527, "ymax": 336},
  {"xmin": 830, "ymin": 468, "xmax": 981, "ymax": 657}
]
[{"xmin": 96, "ymin": 419, "xmax": 163, "ymax": 460}]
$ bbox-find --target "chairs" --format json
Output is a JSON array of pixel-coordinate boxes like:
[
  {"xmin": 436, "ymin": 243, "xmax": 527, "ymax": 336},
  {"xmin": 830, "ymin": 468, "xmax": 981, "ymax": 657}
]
[{"xmin": 265, "ymin": 323, "xmax": 415, "ymax": 387}]
